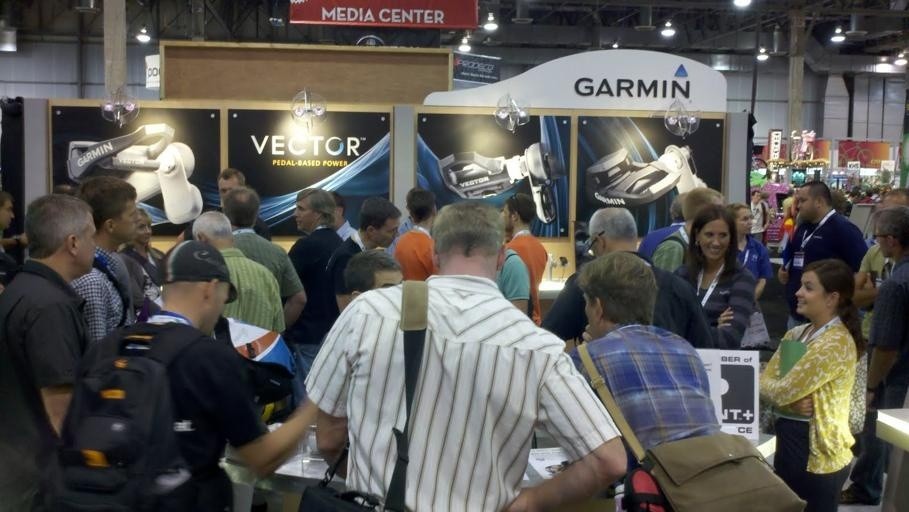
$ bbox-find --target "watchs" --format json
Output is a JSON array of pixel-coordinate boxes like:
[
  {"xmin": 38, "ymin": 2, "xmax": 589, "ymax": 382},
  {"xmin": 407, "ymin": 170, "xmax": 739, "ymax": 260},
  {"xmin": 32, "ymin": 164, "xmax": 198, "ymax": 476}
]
[{"xmin": 11, "ymin": 235, "xmax": 23, "ymax": 246}]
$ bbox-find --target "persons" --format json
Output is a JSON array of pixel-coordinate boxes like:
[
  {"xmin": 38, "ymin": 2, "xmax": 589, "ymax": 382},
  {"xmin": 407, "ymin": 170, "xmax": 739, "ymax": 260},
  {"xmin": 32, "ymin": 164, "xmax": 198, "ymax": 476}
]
[
  {"xmin": 210, "ymin": 169, "xmax": 271, "ymax": 241},
  {"xmin": 0, "ymin": 193, "xmax": 96, "ymax": 512},
  {"xmin": 395, "ymin": 186, "xmax": 437, "ymax": 280},
  {"xmin": 342, "ymin": 246, "xmax": 403, "ymax": 303},
  {"xmin": 725, "ymin": 202, "xmax": 773, "ymax": 306},
  {"xmin": 749, "ymin": 189, "xmax": 769, "ymax": 247},
  {"xmin": 62, "ymin": 240, "xmax": 319, "ymax": 512},
  {"xmin": 674, "ymin": 203, "xmax": 755, "ymax": 350},
  {"xmin": 851, "ymin": 188, "xmax": 909, "ymax": 366},
  {"xmin": 651, "ymin": 187, "xmax": 724, "ymax": 274},
  {"xmin": 567, "ymin": 250, "xmax": 722, "ymax": 512},
  {"xmin": 357, "ymin": 196, "xmax": 403, "ymax": 252},
  {"xmin": 220, "ymin": 187, "xmax": 308, "ymax": 337},
  {"xmin": 501, "ymin": 192, "xmax": 550, "ymax": 324},
  {"xmin": 287, "ymin": 190, "xmax": 344, "ymax": 343},
  {"xmin": 118, "ymin": 209, "xmax": 168, "ymax": 290},
  {"xmin": 328, "ymin": 186, "xmax": 362, "ymax": 252},
  {"xmin": 540, "ymin": 208, "xmax": 716, "ymax": 351},
  {"xmin": 777, "ymin": 180, "xmax": 867, "ymax": 328},
  {"xmin": 192, "ymin": 211, "xmax": 288, "ymax": 339},
  {"xmin": 492, "ymin": 237, "xmax": 531, "ymax": 317},
  {"xmin": 303, "ymin": 201, "xmax": 628, "ymax": 512},
  {"xmin": 838, "ymin": 204, "xmax": 909, "ymax": 506},
  {"xmin": 68, "ymin": 174, "xmax": 142, "ymax": 347},
  {"xmin": 0, "ymin": 190, "xmax": 29, "ymax": 291},
  {"xmin": 638, "ymin": 193, "xmax": 685, "ymax": 262},
  {"xmin": 760, "ymin": 258, "xmax": 857, "ymax": 512}
]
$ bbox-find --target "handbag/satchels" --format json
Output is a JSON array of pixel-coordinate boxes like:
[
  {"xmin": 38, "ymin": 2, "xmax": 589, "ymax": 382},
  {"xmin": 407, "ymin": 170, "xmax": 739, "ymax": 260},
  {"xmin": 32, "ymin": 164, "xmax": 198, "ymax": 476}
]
[
  {"xmin": 577, "ymin": 343, "xmax": 808, "ymax": 512},
  {"xmin": 297, "ymin": 281, "xmax": 430, "ymax": 512}
]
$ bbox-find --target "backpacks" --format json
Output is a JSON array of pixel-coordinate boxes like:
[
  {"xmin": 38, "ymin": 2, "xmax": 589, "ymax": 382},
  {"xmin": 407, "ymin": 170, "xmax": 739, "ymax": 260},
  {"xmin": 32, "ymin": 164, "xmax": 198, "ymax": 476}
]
[{"xmin": 35, "ymin": 321, "xmax": 207, "ymax": 512}]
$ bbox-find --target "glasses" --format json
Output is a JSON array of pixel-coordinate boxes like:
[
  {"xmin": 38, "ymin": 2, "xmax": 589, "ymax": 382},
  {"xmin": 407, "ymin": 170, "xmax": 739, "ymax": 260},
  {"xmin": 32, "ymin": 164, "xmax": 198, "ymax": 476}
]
[
  {"xmin": 872, "ymin": 233, "xmax": 897, "ymax": 242},
  {"xmin": 585, "ymin": 232, "xmax": 605, "ymax": 251}
]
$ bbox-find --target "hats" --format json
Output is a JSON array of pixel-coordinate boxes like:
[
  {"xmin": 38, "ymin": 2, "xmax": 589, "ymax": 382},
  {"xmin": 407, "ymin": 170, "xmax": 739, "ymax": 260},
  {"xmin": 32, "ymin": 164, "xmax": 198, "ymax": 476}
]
[{"xmin": 160, "ymin": 239, "xmax": 239, "ymax": 305}]
